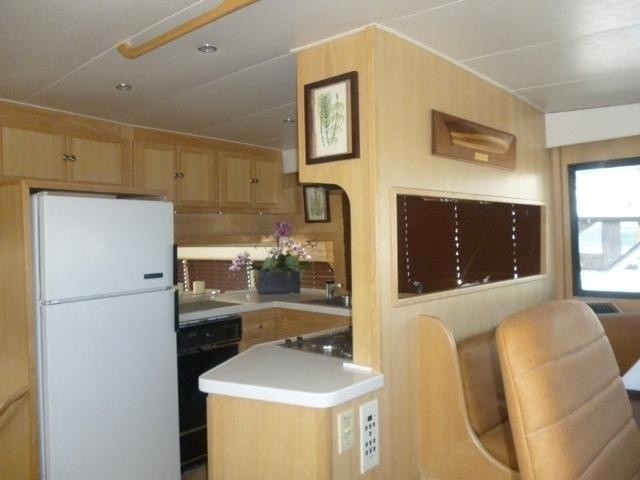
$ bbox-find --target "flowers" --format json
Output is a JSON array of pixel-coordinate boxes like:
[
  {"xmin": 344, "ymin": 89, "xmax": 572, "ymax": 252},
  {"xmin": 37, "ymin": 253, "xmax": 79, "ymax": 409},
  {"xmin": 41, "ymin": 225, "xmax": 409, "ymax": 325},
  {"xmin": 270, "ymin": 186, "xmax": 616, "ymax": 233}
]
[{"xmin": 224, "ymin": 219, "xmax": 314, "ymax": 276}]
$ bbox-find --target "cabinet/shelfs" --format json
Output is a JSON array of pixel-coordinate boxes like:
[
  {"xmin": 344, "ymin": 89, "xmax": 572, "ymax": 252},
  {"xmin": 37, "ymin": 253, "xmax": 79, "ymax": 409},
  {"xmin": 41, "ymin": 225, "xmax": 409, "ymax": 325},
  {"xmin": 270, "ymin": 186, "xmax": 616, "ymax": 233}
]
[
  {"xmin": 276, "ymin": 305, "xmax": 353, "ymax": 343},
  {"xmin": 132, "ymin": 125, "xmax": 219, "ymax": 215},
  {"xmin": 0, "ymin": 100, "xmax": 132, "ymax": 198},
  {"xmin": 219, "ymin": 139, "xmax": 291, "ymax": 217},
  {"xmin": 1, "ymin": 176, "xmax": 43, "ymax": 478},
  {"xmin": 234, "ymin": 308, "xmax": 276, "ymax": 350}
]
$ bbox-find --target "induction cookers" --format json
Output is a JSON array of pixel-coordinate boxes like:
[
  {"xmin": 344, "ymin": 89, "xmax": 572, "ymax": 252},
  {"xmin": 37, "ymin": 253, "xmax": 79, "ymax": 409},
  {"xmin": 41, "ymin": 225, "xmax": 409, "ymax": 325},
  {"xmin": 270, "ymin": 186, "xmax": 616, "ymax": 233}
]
[{"xmin": 177, "ymin": 299, "xmax": 244, "ymax": 344}]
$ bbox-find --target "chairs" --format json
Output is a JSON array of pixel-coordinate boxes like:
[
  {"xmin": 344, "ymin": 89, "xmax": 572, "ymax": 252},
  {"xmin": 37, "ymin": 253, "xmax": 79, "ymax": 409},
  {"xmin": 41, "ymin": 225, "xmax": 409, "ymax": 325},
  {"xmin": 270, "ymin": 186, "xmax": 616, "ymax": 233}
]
[{"xmin": 491, "ymin": 294, "xmax": 639, "ymax": 480}]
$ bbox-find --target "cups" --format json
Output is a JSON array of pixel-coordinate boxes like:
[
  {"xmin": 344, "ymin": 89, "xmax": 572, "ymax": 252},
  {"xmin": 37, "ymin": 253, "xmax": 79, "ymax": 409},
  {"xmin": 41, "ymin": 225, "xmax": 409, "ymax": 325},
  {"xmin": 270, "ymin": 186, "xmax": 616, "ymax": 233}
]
[{"xmin": 192, "ymin": 281, "xmax": 205, "ymax": 294}]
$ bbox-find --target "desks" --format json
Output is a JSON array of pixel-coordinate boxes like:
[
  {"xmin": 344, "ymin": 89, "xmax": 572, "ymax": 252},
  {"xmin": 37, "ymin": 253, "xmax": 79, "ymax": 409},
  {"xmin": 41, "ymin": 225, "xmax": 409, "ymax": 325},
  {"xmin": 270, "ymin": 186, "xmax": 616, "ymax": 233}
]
[{"xmin": 621, "ymin": 358, "xmax": 639, "ymax": 399}]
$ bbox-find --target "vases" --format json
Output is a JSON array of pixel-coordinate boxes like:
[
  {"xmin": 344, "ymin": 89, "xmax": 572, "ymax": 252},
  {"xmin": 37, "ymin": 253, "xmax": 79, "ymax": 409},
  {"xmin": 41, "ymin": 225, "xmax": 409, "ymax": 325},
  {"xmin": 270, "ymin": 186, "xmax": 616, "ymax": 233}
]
[{"xmin": 253, "ymin": 268, "xmax": 302, "ymax": 294}]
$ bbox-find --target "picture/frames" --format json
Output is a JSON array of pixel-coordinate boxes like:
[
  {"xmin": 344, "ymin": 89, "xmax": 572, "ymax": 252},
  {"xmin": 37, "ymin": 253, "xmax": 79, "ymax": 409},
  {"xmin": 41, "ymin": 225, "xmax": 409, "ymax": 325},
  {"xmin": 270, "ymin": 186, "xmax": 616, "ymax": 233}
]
[
  {"xmin": 302, "ymin": 69, "xmax": 359, "ymax": 167},
  {"xmin": 302, "ymin": 182, "xmax": 331, "ymax": 224}
]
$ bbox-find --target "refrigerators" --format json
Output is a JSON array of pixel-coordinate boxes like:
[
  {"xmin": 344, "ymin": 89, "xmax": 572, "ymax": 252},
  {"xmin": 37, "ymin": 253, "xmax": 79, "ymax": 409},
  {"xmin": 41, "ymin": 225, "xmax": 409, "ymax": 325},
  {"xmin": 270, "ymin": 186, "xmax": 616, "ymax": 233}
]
[{"xmin": 35, "ymin": 192, "xmax": 185, "ymax": 480}]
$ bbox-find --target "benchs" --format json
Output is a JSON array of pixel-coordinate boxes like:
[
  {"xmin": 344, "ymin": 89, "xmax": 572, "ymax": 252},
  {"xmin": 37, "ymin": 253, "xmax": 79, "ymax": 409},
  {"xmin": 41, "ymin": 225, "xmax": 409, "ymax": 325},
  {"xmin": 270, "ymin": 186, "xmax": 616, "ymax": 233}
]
[{"xmin": 408, "ymin": 301, "xmax": 639, "ymax": 479}]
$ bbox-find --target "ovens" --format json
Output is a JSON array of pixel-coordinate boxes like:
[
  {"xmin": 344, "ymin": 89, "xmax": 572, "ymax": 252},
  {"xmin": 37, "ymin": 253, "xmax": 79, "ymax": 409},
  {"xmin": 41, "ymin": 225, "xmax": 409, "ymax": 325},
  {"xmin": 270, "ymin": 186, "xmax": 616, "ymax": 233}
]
[{"xmin": 176, "ymin": 343, "xmax": 239, "ymax": 472}]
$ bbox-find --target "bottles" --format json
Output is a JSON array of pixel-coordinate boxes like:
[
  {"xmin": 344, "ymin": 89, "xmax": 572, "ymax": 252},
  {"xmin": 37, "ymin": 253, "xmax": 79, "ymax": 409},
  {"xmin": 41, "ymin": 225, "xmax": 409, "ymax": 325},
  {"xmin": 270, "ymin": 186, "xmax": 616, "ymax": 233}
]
[{"xmin": 326, "ymin": 279, "xmax": 336, "ymax": 299}]
